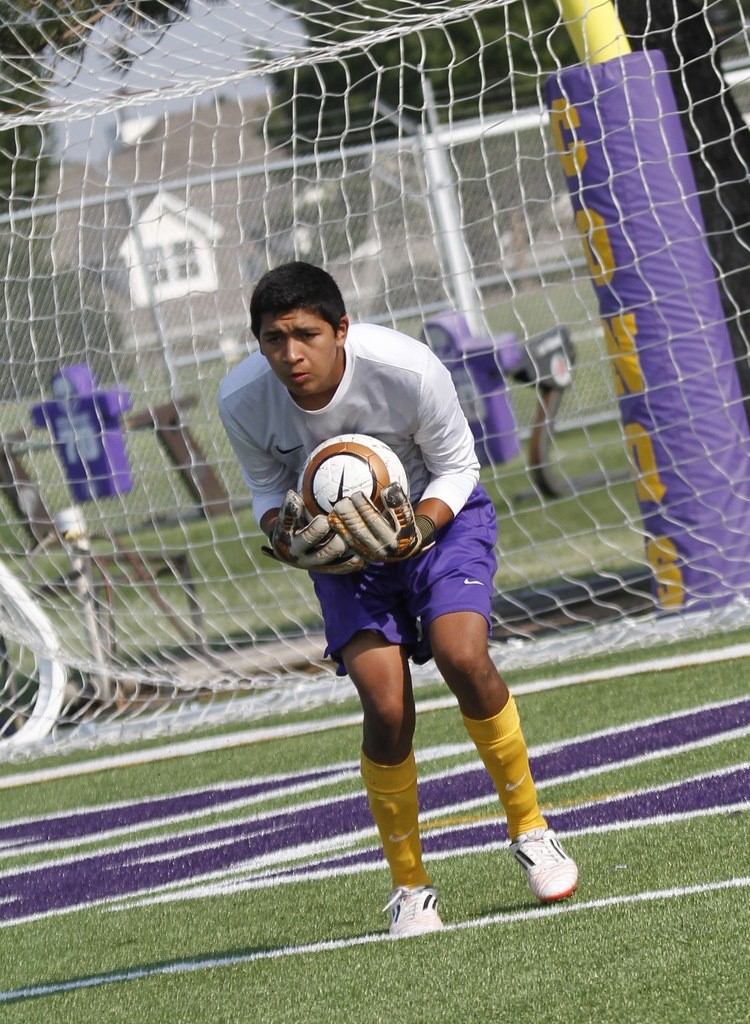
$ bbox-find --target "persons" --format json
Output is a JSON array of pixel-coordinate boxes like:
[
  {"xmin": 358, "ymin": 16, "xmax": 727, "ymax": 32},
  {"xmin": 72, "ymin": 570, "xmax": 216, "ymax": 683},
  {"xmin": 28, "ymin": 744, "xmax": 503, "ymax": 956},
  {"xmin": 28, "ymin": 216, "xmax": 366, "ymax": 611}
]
[{"xmin": 218, "ymin": 262, "xmax": 580, "ymax": 935}]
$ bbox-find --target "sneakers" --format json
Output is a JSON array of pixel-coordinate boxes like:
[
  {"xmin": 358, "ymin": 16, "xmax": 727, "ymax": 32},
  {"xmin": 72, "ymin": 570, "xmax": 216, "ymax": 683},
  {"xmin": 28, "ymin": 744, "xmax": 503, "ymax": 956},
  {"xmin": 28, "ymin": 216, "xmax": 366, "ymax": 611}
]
[
  {"xmin": 509, "ymin": 828, "xmax": 578, "ymax": 901},
  {"xmin": 381, "ymin": 885, "xmax": 444, "ymax": 937}
]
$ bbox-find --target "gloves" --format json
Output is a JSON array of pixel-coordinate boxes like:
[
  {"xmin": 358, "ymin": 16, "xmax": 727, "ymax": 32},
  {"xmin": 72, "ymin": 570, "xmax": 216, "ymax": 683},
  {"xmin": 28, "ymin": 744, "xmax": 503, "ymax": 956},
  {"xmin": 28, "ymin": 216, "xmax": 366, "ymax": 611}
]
[
  {"xmin": 260, "ymin": 490, "xmax": 364, "ymax": 575},
  {"xmin": 327, "ymin": 482, "xmax": 436, "ymax": 563}
]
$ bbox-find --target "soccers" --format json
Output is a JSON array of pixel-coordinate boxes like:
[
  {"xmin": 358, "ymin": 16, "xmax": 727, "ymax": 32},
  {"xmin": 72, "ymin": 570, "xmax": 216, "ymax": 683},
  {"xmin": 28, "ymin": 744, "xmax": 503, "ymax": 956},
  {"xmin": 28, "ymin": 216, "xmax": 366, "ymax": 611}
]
[{"xmin": 296, "ymin": 432, "xmax": 412, "ymax": 559}]
